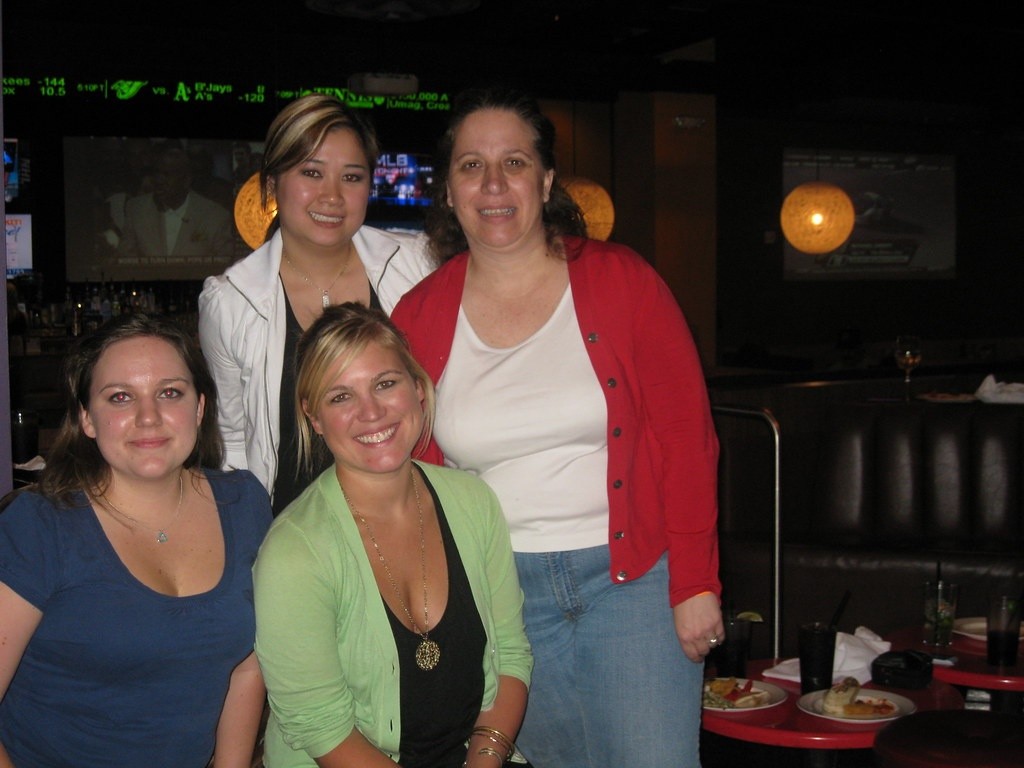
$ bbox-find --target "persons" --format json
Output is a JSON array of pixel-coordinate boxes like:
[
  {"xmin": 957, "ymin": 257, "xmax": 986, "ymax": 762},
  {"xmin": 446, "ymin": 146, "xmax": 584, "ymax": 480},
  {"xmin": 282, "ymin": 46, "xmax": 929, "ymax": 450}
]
[
  {"xmin": 390, "ymin": 86, "xmax": 724, "ymax": 768},
  {"xmin": 252, "ymin": 300, "xmax": 533, "ymax": 768},
  {"xmin": 0, "ymin": 309, "xmax": 274, "ymax": 768},
  {"xmin": 198, "ymin": 97, "xmax": 446, "ymax": 518},
  {"xmin": 113, "ymin": 142, "xmax": 265, "ymax": 279},
  {"xmin": 7, "ymin": 283, "xmax": 30, "ymax": 363}
]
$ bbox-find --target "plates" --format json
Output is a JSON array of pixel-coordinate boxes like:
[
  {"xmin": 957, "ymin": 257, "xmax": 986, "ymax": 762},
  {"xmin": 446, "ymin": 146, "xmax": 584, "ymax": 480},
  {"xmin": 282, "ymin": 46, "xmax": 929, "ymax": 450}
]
[
  {"xmin": 702, "ymin": 678, "xmax": 787, "ymax": 712},
  {"xmin": 796, "ymin": 688, "xmax": 917, "ymax": 724},
  {"xmin": 917, "ymin": 392, "xmax": 977, "ymax": 403},
  {"xmin": 952, "ymin": 616, "xmax": 1024, "ymax": 642}
]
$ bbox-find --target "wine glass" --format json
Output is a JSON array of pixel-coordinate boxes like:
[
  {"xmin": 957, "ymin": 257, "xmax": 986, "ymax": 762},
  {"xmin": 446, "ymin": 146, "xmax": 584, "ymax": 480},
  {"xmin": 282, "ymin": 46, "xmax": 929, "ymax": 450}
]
[{"xmin": 895, "ymin": 335, "xmax": 922, "ymax": 386}]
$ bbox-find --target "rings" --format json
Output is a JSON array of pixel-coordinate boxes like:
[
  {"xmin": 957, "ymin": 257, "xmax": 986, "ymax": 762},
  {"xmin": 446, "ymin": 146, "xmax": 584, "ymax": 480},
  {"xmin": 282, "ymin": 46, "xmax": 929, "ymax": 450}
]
[{"xmin": 707, "ymin": 636, "xmax": 718, "ymax": 644}]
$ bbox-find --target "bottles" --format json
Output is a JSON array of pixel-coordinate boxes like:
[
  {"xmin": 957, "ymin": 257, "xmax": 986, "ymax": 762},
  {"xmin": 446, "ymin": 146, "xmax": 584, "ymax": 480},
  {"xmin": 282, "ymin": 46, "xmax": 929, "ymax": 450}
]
[{"xmin": 20, "ymin": 282, "xmax": 192, "ymax": 338}]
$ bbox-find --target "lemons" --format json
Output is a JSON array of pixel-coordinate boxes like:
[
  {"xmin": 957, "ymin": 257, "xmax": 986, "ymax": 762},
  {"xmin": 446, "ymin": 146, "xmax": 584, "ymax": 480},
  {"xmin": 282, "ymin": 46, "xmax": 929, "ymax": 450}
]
[{"xmin": 736, "ymin": 610, "xmax": 764, "ymax": 622}]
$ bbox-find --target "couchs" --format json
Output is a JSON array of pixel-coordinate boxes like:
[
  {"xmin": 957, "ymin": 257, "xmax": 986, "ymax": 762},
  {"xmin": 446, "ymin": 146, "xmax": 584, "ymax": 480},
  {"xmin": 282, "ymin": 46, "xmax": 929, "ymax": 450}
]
[{"xmin": 717, "ymin": 394, "xmax": 1024, "ymax": 660}]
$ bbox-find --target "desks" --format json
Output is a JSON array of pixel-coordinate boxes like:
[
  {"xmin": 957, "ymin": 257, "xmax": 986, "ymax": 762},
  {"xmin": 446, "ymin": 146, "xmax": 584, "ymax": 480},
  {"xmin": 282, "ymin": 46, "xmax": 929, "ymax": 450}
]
[{"xmin": 699, "ymin": 612, "xmax": 1024, "ymax": 768}]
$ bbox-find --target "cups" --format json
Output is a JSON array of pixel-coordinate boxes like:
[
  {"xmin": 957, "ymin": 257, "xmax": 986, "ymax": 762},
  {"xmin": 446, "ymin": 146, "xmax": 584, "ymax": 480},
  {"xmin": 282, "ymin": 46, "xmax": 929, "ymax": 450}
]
[
  {"xmin": 986, "ymin": 595, "xmax": 1021, "ymax": 668},
  {"xmin": 797, "ymin": 621, "xmax": 838, "ymax": 693},
  {"xmin": 921, "ymin": 582, "xmax": 956, "ymax": 646}
]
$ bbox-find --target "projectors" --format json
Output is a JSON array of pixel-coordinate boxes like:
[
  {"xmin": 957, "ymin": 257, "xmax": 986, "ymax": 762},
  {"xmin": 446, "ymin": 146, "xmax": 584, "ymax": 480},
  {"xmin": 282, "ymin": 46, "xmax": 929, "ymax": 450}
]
[{"xmin": 347, "ymin": 73, "xmax": 419, "ymax": 94}]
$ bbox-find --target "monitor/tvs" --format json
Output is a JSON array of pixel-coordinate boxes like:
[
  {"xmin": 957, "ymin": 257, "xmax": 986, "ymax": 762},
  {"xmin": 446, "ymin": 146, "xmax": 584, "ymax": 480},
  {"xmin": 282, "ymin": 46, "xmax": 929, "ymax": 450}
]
[
  {"xmin": 3, "ymin": 138, "xmax": 18, "ymax": 202},
  {"xmin": 366, "ymin": 151, "xmax": 440, "ymax": 215}
]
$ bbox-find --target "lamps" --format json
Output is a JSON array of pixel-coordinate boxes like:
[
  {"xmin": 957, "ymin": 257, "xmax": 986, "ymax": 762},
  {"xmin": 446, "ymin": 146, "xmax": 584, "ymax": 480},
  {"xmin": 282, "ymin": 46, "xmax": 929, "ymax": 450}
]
[
  {"xmin": 780, "ymin": 146, "xmax": 855, "ymax": 255},
  {"xmin": 232, "ymin": 172, "xmax": 280, "ymax": 251},
  {"xmin": 555, "ymin": 103, "xmax": 615, "ymax": 241}
]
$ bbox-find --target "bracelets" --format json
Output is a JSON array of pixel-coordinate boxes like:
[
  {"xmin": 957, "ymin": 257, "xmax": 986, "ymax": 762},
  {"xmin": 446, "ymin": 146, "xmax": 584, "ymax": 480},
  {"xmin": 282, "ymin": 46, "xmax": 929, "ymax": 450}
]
[{"xmin": 462, "ymin": 726, "xmax": 515, "ymax": 768}]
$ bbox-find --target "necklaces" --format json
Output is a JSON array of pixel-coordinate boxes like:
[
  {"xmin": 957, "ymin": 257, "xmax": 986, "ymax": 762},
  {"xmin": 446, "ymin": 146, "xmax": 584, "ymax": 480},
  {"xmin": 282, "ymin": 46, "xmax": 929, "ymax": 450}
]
[
  {"xmin": 336, "ymin": 465, "xmax": 440, "ymax": 671},
  {"xmin": 282, "ymin": 241, "xmax": 352, "ymax": 309},
  {"xmin": 97, "ymin": 477, "xmax": 182, "ymax": 544}
]
